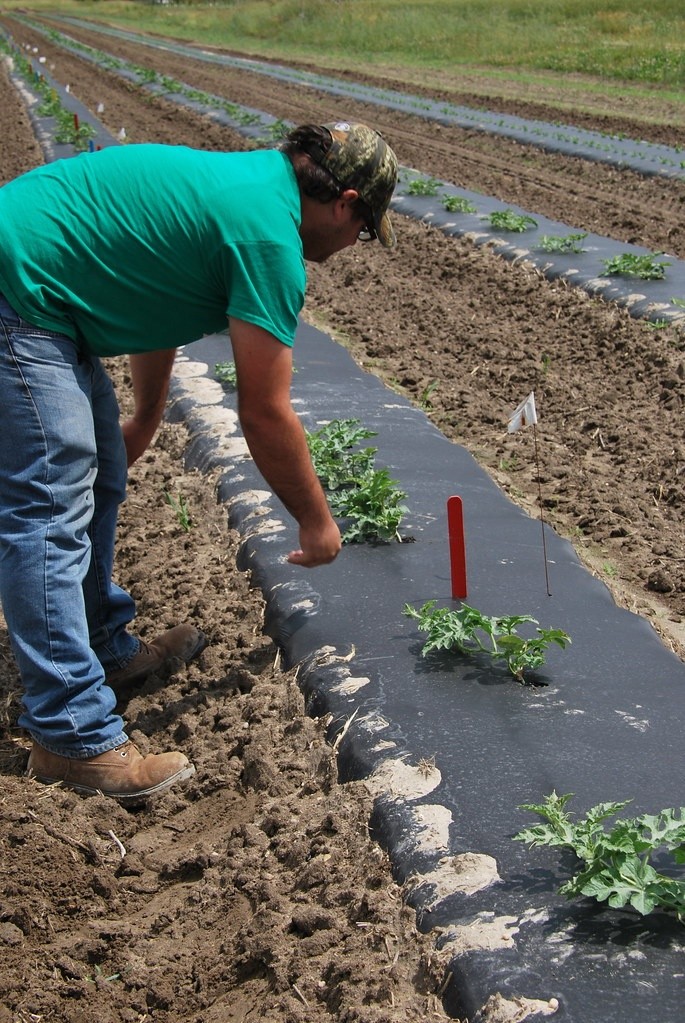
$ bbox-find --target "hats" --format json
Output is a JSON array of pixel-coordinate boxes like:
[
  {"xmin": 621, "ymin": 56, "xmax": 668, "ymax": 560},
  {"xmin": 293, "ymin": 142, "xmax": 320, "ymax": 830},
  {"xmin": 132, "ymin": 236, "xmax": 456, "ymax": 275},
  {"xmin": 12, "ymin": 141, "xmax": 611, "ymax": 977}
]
[{"xmin": 289, "ymin": 121, "xmax": 397, "ymax": 247}]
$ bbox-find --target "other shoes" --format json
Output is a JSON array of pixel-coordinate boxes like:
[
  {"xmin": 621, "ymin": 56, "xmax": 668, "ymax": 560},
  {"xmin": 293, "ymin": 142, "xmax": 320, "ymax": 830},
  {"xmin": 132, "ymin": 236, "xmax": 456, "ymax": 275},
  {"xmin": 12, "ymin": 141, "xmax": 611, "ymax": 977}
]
[
  {"xmin": 23, "ymin": 740, "xmax": 196, "ymax": 808},
  {"xmin": 104, "ymin": 624, "xmax": 206, "ymax": 689}
]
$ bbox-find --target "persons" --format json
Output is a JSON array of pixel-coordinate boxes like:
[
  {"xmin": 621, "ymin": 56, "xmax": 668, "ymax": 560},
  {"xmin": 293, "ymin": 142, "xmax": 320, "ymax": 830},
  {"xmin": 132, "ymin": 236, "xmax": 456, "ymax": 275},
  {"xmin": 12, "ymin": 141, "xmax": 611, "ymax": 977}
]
[{"xmin": 0, "ymin": 119, "xmax": 401, "ymax": 807}]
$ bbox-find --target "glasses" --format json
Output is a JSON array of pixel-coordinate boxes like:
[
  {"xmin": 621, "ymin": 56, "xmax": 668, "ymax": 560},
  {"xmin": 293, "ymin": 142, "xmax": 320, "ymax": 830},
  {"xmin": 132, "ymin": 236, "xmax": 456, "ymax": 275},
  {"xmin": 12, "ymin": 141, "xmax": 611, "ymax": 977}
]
[{"xmin": 340, "ymin": 186, "xmax": 377, "ymax": 241}]
